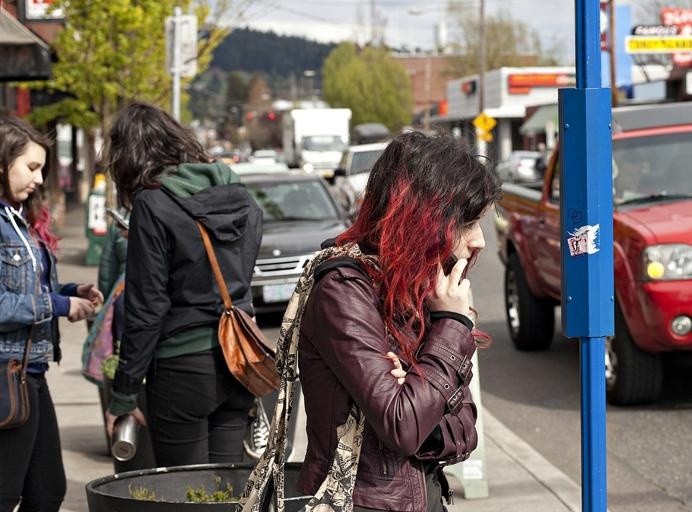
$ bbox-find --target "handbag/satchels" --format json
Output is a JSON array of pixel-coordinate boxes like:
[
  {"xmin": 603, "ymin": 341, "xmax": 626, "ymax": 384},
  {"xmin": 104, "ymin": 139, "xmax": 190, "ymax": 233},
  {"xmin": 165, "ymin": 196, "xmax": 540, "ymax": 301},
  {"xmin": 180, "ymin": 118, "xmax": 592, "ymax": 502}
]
[
  {"xmin": 0, "ymin": 357, "xmax": 30, "ymax": 428},
  {"xmin": 218, "ymin": 306, "xmax": 281, "ymax": 397}
]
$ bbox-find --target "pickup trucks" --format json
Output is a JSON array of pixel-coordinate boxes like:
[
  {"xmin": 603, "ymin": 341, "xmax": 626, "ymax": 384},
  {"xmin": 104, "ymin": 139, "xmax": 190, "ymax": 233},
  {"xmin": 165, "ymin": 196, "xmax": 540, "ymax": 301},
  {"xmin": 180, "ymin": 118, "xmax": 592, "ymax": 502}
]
[{"xmin": 489, "ymin": 99, "xmax": 692, "ymax": 408}]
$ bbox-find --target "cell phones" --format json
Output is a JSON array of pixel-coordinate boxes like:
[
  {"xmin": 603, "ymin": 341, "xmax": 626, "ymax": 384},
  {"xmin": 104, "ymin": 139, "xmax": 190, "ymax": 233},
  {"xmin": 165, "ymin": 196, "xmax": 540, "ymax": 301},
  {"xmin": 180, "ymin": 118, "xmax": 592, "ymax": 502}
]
[
  {"xmin": 106, "ymin": 209, "xmax": 130, "ymax": 230},
  {"xmin": 443, "ymin": 254, "xmax": 460, "ymax": 285}
]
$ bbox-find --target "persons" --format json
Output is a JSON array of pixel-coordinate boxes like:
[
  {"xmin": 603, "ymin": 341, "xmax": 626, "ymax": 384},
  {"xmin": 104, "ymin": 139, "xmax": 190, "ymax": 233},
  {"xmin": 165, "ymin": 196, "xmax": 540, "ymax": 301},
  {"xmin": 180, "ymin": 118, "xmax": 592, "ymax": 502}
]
[
  {"xmin": 100, "ymin": 102, "xmax": 264, "ymax": 470},
  {"xmin": 298, "ymin": 130, "xmax": 503, "ymax": 512},
  {"xmin": 0, "ymin": 116, "xmax": 107, "ymax": 511}
]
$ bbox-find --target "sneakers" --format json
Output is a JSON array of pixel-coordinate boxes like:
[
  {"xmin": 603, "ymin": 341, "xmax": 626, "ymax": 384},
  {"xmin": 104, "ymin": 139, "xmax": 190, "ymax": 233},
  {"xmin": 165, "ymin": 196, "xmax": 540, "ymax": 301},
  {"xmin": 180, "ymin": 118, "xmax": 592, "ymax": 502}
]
[{"xmin": 243, "ymin": 399, "xmax": 270, "ymax": 457}]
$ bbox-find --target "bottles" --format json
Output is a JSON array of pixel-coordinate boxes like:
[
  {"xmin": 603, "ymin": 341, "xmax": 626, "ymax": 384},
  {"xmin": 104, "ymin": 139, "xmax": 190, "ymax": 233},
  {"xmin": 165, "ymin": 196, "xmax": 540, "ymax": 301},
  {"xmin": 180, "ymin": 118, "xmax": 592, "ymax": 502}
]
[{"xmin": 110, "ymin": 413, "xmax": 142, "ymax": 462}]
